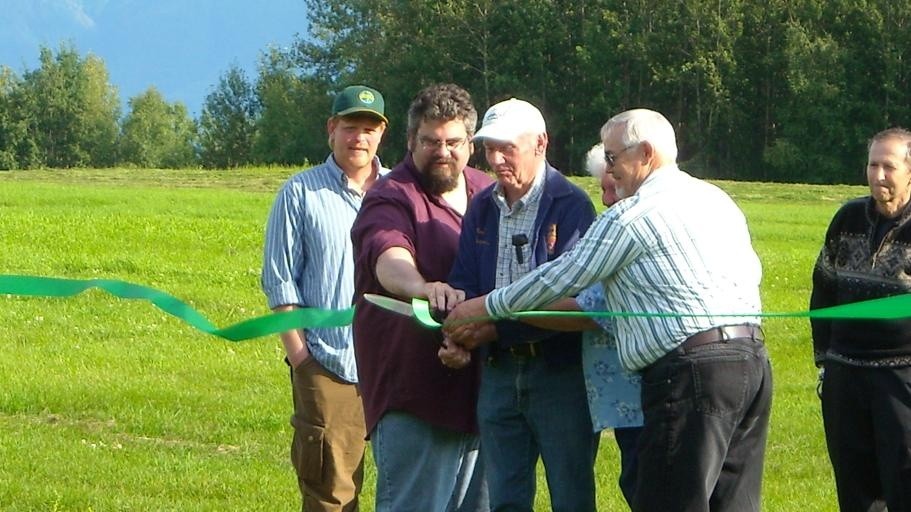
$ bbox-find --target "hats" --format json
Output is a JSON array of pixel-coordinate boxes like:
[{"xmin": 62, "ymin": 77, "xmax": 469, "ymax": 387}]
[
  {"xmin": 332, "ymin": 85, "xmax": 388, "ymax": 124},
  {"xmin": 469, "ymin": 98, "xmax": 546, "ymax": 144}
]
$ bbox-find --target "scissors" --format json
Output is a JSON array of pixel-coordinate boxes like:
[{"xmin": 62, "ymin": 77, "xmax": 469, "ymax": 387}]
[{"xmin": 362, "ymin": 294, "xmax": 449, "ymax": 350}]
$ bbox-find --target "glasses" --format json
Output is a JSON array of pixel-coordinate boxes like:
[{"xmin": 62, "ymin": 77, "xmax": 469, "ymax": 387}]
[
  {"xmin": 605, "ymin": 145, "xmax": 640, "ymax": 167},
  {"xmin": 416, "ymin": 134, "xmax": 466, "ymax": 151}
]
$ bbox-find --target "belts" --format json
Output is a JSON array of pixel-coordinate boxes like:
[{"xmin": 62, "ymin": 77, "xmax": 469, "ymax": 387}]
[
  {"xmin": 494, "ymin": 341, "xmax": 551, "ymax": 354},
  {"xmin": 684, "ymin": 326, "xmax": 764, "ymax": 350}
]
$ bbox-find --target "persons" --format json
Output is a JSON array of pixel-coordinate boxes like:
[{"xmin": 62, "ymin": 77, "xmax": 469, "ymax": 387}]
[
  {"xmin": 808, "ymin": 125, "xmax": 911, "ymax": 511},
  {"xmin": 260, "ymin": 84, "xmax": 393, "ymax": 511},
  {"xmin": 515, "ymin": 140, "xmax": 647, "ymax": 510},
  {"xmin": 435, "ymin": 96, "xmax": 599, "ymax": 512},
  {"xmin": 349, "ymin": 81, "xmax": 496, "ymax": 511},
  {"xmin": 442, "ymin": 107, "xmax": 773, "ymax": 511}
]
